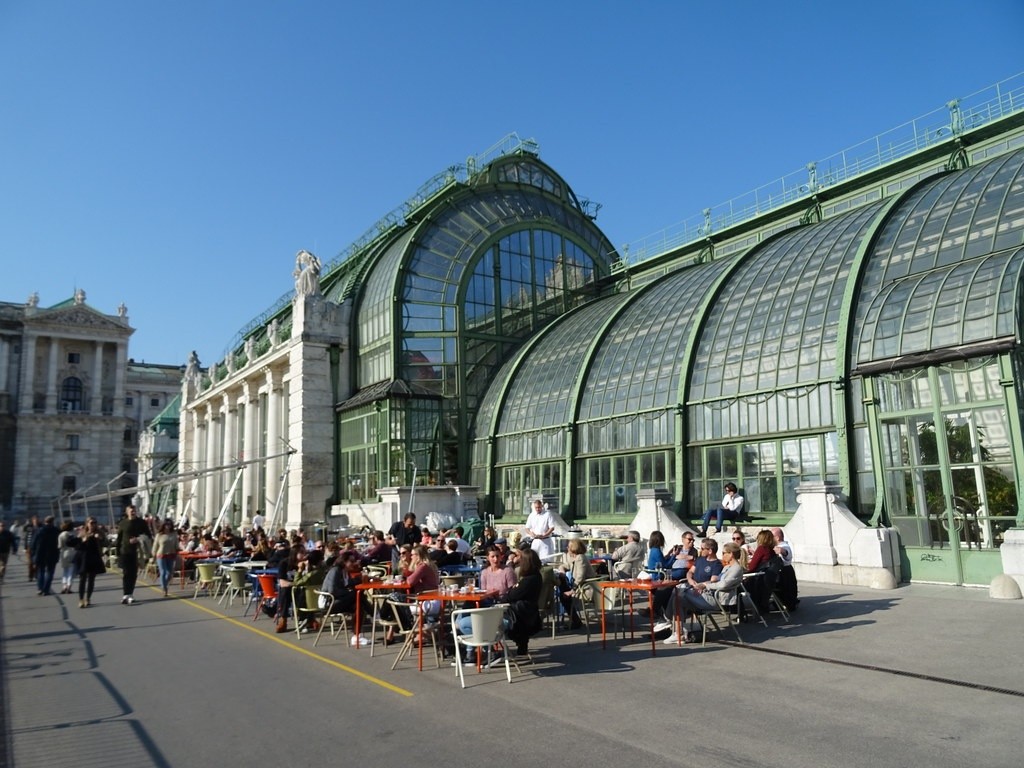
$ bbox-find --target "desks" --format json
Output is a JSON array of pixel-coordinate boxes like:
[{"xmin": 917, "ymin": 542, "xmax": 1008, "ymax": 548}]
[
  {"xmin": 354, "ymin": 579, "xmax": 412, "ymax": 651},
  {"xmin": 231, "ymin": 561, "xmax": 268, "ymax": 605},
  {"xmin": 254, "ymin": 568, "xmax": 280, "ymax": 626},
  {"xmin": 598, "ymin": 577, "xmax": 679, "ymax": 656},
  {"xmin": 415, "ymin": 589, "xmax": 503, "ymax": 675},
  {"xmin": 179, "ymin": 550, "xmax": 224, "ymax": 588}
]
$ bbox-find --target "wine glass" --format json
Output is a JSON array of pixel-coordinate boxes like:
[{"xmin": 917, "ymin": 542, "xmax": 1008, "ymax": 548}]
[{"xmin": 655, "ymin": 561, "xmax": 663, "ymax": 582}]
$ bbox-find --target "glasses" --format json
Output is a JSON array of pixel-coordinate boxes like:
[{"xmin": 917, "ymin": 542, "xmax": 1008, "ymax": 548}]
[
  {"xmin": 436, "ymin": 540, "xmax": 445, "ymax": 542},
  {"xmin": 732, "ymin": 537, "xmax": 741, "ymax": 541},
  {"xmin": 685, "ymin": 538, "xmax": 695, "ymax": 541},
  {"xmin": 701, "ymin": 547, "xmax": 709, "ymax": 551},
  {"xmin": 398, "ymin": 551, "xmax": 408, "ymax": 556},
  {"xmin": 722, "ymin": 551, "xmax": 732, "ymax": 555},
  {"xmin": 350, "ymin": 559, "xmax": 356, "ymax": 564},
  {"xmin": 725, "ymin": 490, "xmax": 732, "ymax": 492}
]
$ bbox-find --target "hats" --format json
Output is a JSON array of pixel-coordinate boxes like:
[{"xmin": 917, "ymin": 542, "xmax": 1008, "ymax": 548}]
[{"xmin": 493, "ymin": 537, "xmax": 507, "ymax": 545}]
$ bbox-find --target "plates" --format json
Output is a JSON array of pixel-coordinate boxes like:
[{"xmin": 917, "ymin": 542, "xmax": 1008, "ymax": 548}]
[{"xmin": 475, "ymin": 590, "xmax": 487, "ymax": 593}]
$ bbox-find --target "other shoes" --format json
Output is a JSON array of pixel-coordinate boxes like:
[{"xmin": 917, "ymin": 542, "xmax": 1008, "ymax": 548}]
[
  {"xmin": 450, "ymin": 656, "xmax": 477, "ymax": 667},
  {"xmin": 483, "ymin": 651, "xmax": 502, "ymax": 669},
  {"xmin": 383, "ymin": 636, "xmax": 396, "ymax": 645},
  {"xmin": 26, "ymin": 575, "xmax": 169, "ymax": 608},
  {"xmin": 696, "ymin": 531, "xmax": 707, "ymax": 538},
  {"xmin": 714, "ymin": 526, "xmax": 722, "ymax": 533},
  {"xmin": 276, "ymin": 616, "xmax": 287, "ymax": 633},
  {"xmin": 640, "ymin": 605, "xmax": 687, "ymax": 644},
  {"xmin": 350, "ymin": 635, "xmax": 368, "ymax": 646}
]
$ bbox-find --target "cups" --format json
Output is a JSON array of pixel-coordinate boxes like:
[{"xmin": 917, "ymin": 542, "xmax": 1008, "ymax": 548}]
[
  {"xmin": 632, "ymin": 568, "xmax": 637, "ymax": 579},
  {"xmin": 438, "ymin": 584, "xmax": 467, "ymax": 596},
  {"xmin": 589, "ymin": 548, "xmax": 594, "ymax": 557},
  {"xmin": 395, "ymin": 575, "xmax": 402, "ymax": 581},
  {"xmin": 468, "ymin": 578, "xmax": 475, "ymax": 593},
  {"xmin": 468, "ymin": 560, "xmax": 472, "ymax": 567},
  {"xmin": 387, "ymin": 575, "xmax": 393, "ymax": 581},
  {"xmin": 598, "ymin": 548, "xmax": 603, "ymax": 555},
  {"xmin": 479, "ymin": 559, "xmax": 483, "ymax": 566}
]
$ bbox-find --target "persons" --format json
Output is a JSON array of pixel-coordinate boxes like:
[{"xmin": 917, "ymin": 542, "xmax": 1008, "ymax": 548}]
[
  {"xmin": 1, "ymin": 499, "xmax": 791, "ymax": 667},
  {"xmin": 697, "ymin": 483, "xmax": 744, "ymax": 537}
]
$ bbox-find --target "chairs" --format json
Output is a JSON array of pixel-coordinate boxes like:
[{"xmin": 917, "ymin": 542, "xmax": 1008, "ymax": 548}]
[{"xmin": 98, "ymin": 513, "xmax": 790, "ymax": 689}]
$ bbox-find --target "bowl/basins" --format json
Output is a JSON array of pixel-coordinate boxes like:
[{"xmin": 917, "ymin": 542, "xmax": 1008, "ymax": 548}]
[{"xmin": 439, "ymin": 576, "xmax": 467, "ymax": 588}]
